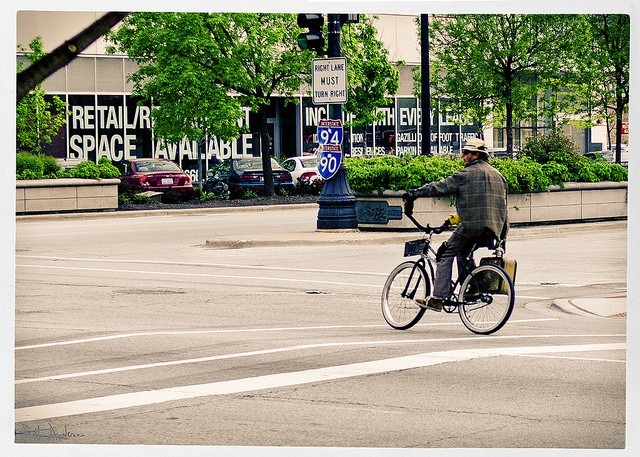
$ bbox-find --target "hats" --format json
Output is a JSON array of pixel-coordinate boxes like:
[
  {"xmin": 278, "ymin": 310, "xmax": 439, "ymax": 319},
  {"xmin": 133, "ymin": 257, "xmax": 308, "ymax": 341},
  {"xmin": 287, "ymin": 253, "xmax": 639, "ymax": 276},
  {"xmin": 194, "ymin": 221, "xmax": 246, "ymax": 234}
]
[{"xmin": 462, "ymin": 138, "xmax": 490, "ymax": 157}]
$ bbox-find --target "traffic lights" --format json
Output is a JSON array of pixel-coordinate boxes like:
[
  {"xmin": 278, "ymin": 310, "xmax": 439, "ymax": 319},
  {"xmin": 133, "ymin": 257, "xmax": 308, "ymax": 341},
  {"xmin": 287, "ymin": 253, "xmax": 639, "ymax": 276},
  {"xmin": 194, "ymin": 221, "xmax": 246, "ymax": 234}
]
[{"xmin": 297, "ymin": 14, "xmax": 325, "ymax": 50}]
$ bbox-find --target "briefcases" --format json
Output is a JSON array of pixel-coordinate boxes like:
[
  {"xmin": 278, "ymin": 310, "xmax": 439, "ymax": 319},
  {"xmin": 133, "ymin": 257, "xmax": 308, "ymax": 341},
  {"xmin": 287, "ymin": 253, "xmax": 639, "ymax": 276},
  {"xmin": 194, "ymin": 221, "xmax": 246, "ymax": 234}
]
[{"xmin": 478, "ymin": 255, "xmax": 517, "ymax": 295}]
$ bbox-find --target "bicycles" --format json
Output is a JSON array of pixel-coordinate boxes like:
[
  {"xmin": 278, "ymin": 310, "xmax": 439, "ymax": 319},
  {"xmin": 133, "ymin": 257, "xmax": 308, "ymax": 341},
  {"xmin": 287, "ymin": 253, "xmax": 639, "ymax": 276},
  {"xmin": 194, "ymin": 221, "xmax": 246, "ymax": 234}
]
[{"xmin": 382, "ymin": 202, "xmax": 515, "ymax": 335}]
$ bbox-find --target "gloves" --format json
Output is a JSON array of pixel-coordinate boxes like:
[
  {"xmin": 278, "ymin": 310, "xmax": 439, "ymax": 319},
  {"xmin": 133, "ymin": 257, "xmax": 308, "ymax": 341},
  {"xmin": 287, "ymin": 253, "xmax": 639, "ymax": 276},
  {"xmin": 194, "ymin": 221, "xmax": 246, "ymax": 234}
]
[
  {"xmin": 402, "ymin": 192, "xmax": 418, "ymax": 203},
  {"xmin": 492, "ymin": 240, "xmax": 505, "ymax": 254}
]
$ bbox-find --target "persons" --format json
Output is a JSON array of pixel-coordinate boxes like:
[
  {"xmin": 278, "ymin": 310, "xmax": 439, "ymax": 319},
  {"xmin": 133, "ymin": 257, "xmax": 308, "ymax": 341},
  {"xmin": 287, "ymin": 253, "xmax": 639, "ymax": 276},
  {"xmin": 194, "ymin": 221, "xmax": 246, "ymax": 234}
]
[{"xmin": 402, "ymin": 137, "xmax": 509, "ymax": 312}]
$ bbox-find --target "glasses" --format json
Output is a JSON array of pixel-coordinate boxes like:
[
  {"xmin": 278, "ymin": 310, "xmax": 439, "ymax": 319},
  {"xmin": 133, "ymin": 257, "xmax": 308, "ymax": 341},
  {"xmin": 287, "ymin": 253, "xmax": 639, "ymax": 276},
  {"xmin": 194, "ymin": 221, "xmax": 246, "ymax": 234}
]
[{"xmin": 462, "ymin": 149, "xmax": 470, "ymax": 155}]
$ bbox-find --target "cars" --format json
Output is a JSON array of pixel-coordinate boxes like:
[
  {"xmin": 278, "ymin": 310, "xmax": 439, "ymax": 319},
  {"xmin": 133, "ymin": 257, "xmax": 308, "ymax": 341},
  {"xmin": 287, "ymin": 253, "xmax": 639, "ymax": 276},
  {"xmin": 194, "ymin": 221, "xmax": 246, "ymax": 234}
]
[
  {"xmin": 230, "ymin": 157, "xmax": 294, "ymax": 198},
  {"xmin": 118, "ymin": 158, "xmax": 193, "ymax": 200},
  {"xmin": 279, "ymin": 156, "xmax": 326, "ymax": 192}
]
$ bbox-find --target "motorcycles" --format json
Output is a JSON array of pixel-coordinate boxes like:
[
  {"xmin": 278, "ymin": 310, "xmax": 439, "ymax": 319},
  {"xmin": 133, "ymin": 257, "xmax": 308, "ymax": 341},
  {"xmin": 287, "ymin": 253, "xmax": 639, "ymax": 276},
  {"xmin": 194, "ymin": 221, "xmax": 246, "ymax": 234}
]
[{"xmin": 203, "ymin": 175, "xmax": 229, "ymax": 195}]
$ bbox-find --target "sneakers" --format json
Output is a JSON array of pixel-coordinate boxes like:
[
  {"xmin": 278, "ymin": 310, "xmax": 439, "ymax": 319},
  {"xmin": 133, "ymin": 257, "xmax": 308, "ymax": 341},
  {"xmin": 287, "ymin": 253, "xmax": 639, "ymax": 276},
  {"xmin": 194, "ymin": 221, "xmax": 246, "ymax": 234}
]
[
  {"xmin": 414, "ymin": 296, "xmax": 443, "ymax": 312},
  {"xmin": 464, "ymin": 287, "xmax": 479, "ymax": 297}
]
